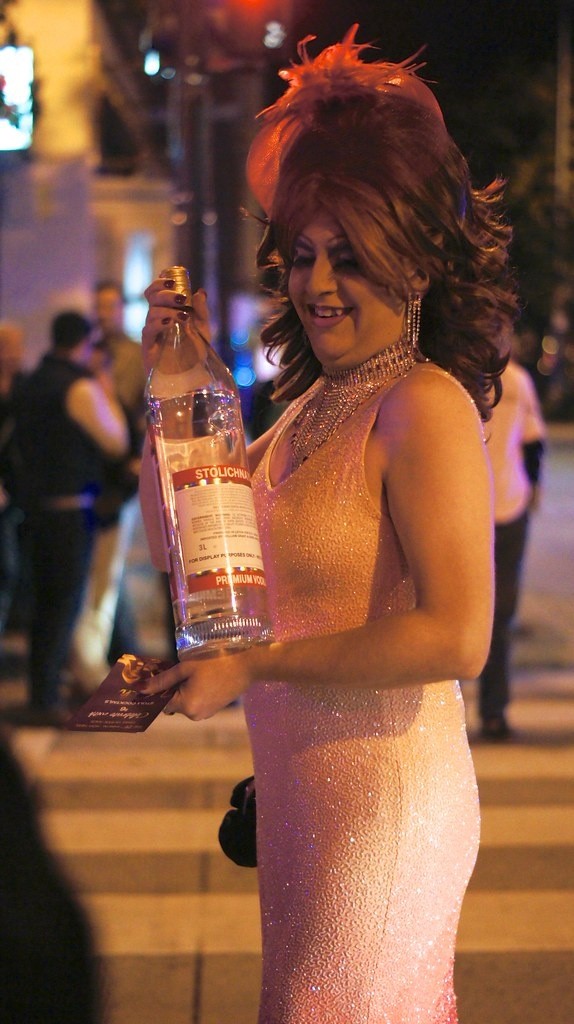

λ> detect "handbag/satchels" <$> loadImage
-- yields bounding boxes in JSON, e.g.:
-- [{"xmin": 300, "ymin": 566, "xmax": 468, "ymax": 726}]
[{"xmin": 218, "ymin": 775, "xmax": 258, "ymax": 868}]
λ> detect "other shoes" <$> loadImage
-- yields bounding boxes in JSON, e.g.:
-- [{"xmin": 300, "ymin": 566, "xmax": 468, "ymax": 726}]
[
  {"xmin": 22, "ymin": 705, "xmax": 73, "ymax": 725},
  {"xmin": 482, "ymin": 718, "xmax": 510, "ymax": 740}
]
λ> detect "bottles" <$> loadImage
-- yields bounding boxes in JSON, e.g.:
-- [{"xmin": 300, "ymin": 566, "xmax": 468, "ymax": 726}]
[{"xmin": 146, "ymin": 264, "xmax": 272, "ymax": 661}]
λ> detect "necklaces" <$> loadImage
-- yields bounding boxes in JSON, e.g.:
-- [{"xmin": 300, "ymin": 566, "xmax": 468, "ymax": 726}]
[{"xmin": 288, "ymin": 337, "xmax": 417, "ymax": 475}]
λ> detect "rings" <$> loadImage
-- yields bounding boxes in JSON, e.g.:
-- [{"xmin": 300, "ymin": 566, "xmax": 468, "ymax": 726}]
[{"xmin": 164, "ymin": 706, "xmax": 175, "ymax": 715}]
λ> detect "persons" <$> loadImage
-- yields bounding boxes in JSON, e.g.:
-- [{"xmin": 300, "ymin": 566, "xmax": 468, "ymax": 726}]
[{"xmin": 0, "ymin": 23, "xmax": 546, "ymax": 1024}]
